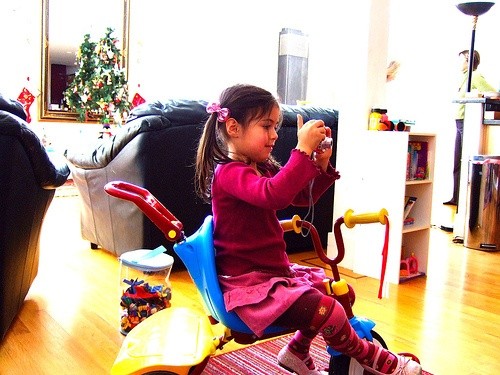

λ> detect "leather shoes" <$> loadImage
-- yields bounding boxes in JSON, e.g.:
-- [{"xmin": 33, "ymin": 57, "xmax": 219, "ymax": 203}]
[
  {"xmin": 361, "ymin": 345, "xmax": 422, "ymax": 375},
  {"xmin": 277, "ymin": 346, "xmax": 323, "ymax": 375}
]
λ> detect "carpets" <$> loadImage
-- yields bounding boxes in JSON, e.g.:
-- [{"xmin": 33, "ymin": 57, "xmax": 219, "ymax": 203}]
[{"xmin": 199, "ymin": 331, "xmax": 331, "ymax": 375}]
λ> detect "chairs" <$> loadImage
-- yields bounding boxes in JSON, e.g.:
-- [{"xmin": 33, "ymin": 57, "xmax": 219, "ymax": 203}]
[{"xmin": 173, "ymin": 215, "xmax": 296, "ymax": 345}]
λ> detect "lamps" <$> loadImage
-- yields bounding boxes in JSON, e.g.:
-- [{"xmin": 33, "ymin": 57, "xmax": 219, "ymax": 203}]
[{"xmin": 456, "ymin": 0, "xmax": 495, "ymax": 96}]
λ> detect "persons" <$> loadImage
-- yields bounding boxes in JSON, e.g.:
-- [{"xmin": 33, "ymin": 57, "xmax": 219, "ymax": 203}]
[
  {"xmin": 195, "ymin": 84, "xmax": 421, "ymax": 375},
  {"xmin": 443, "ymin": 50, "xmax": 497, "ymax": 205}
]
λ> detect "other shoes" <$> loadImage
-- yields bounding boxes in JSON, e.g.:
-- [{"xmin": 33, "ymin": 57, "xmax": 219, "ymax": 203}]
[{"xmin": 443, "ymin": 201, "xmax": 456, "ymax": 205}]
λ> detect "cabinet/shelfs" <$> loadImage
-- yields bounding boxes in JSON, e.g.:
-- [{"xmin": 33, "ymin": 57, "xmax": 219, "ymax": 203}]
[{"xmin": 326, "ymin": 130, "xmax": 437, "ymax": 299}]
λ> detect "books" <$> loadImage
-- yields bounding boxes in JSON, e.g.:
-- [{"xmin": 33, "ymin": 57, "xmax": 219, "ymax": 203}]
[
  {"xmin": 403, "ymin": 195, "xmax": 418, "ymax": 227},
  {"xmin": 406, "ymin": 140, "xmax": 431, "ymax": 181}
]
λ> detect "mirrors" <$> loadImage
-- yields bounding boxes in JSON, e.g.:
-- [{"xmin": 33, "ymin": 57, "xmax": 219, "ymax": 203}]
[{"xmin": 40, "ymin": 0, "xmax": 131, "ymax": 124}]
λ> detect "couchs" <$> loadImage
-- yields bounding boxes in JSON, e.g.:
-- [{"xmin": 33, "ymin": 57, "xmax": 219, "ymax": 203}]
[
  {"xmin": 65, "ymin": 100, "xmax": 340, "ymax": 276},
  {"xmin": 0, "ymin": 96, "xmax": 72, "ymax": 347}
]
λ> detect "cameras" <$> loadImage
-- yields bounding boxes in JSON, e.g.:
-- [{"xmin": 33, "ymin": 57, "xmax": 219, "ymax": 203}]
[{"xmin": 313, "ymin": 136, "xmax": 333, "ymax": 151}]
